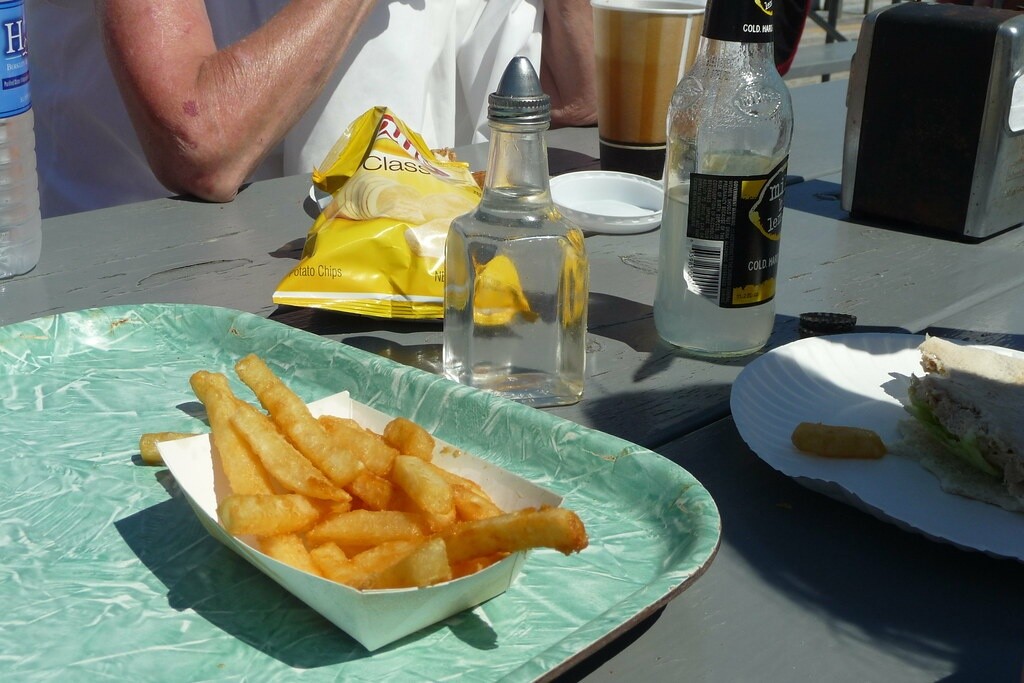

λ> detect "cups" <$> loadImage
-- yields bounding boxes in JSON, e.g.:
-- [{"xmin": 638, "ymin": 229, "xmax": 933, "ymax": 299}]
[{"xmin": 590, "ymin": 0, "xmax": 705, "ymax": 182}]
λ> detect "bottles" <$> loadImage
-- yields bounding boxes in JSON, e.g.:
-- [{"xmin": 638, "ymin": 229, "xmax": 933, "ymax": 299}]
[
  {"xmin": 654, "ymin": 0, "xmax": 792, "ymax": 357},
  {"xmin": 443, "ymin": 56, "xmax": 588, "ymax": 408},
  {"xmin": 0, "ymin": 0, "xmax": 42, "ymax": 278}
]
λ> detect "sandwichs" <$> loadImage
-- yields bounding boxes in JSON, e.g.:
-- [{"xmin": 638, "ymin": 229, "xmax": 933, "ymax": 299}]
[{"xmin": 886, "ymin": 335, "xmax": 1024, "ymax": 514}]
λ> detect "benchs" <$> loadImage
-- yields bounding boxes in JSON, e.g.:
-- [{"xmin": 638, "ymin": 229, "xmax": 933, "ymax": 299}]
[{"xmin": 781, "ymin": 41, "xmax": 858, "ymax": 79}]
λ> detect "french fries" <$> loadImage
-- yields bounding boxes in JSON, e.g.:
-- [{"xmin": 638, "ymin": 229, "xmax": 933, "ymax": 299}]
[
  {"xmin": 791, "ymin": 422, "xmax": 888, "ymax": 458},
  {"xmin": 138, "ymin": 353, "xmax": 589, "ymax": 593}
]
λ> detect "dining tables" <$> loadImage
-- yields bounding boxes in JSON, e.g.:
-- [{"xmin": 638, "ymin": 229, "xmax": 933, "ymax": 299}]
[{"xmin": 0, "ymin": 77, "xmax": 1024, "ymax": 683}]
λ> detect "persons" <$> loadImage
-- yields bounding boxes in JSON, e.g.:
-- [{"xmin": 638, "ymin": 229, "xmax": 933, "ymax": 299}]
[{"xmin": 24, "ymin": 0, "xmax": 597, "ymax": 219}]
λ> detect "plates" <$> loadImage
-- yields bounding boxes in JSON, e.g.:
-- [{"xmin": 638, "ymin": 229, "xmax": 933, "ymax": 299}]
[{"xmin": 729, "ymin": 333, "xmax": 1024, "ymax": 560}]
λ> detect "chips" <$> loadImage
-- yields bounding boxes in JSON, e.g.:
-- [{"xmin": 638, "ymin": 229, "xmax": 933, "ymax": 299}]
[{"xmin": 337, "ymin": 174, "xmax": 477, "ymax": 262}]
